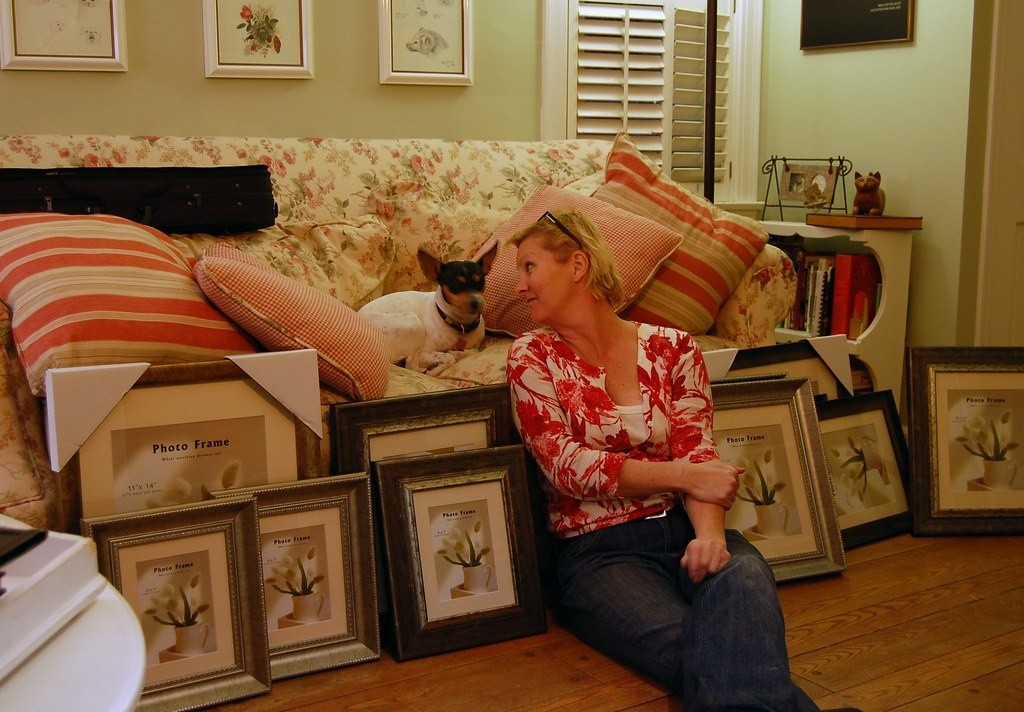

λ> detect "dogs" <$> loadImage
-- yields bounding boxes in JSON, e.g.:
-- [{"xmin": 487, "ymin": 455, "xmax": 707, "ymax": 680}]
[{"xmin": 357, "ymin": 239, "xmax": 499, "ymax": 380}]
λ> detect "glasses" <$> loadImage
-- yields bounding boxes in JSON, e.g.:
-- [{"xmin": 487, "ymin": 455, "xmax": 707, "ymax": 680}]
[{"xmin": 537, "ymin": 210, "xmax": 584, "ymax": 251}]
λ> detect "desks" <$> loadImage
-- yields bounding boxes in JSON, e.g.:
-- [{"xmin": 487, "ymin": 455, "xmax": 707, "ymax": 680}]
[{"xmin": 0, "ymin": 511, "xmax": 148, "ymax": 712}]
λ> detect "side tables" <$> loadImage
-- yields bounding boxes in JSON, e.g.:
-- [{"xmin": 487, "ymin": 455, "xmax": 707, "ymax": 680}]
[{"xmin": 753, "ymin": 214, "xmax": 923, "ymax": 414}]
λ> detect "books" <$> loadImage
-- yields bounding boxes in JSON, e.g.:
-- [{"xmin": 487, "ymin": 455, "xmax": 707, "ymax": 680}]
[{"xmin": 775, "ymin": 250, "xmax": 880, "ymax": 340}]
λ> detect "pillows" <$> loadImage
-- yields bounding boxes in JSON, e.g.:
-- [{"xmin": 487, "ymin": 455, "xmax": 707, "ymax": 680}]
[
  {"xmin": 195, "ymin": 243, "xmax": 387, "ymax": 402},
  {"xmin": 462, "ymin": 186, "xmax": 683, "ymax": 349},
  {"xmin": 0, "ymin": 210, "xmax": 269, "ymax": 419},
  {"xmin": 591, "ymin": 132, "xmax": 769, "ymax": 335}
]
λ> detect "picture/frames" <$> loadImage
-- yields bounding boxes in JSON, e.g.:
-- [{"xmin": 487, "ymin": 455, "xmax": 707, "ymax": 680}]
[
  {"xmin": 800, "ymin": 0, "xmax": 914, "ymax": 50},
  {"xmin": 902, "ymin": 347, "xmax": 1024, "ymax": 538},
  {"xmin": 379, "ymin": 0, "xmax": 474, "ymax": 91},
  {"xmin": 710, "ymin": 378, "xmax": 846, "ymax": 586},
  {"xmin": 0, "ymin": 0, "xmax": 128, "ymax": 73},
  {"xmin": 327, "ymin": 381, "xmax": 543, "ymax": 651},
  {"xmin": 691, "ymin": 334, "xmax": 861, "ymax": 457},
  {"xmin": 203, "ymin": 0, "xmax": 315, "ymax": 81},
  {"xmin": 210, "ymin": 474, "xmax": 380, "ymax": 678},
  {"xmin": 375, "ymin": 444, "xmax": 548, "ymax": 664},
  {"xmin": 816, "ymin": 390, "xmax": 912, "ymax": 544},
  {"xmin": 41, "ymin": 349, "xmax": 318, "ymax": 560},
  {"xmin": 81, "ymin": 492, "xmax": 271, "ymax": 712}
]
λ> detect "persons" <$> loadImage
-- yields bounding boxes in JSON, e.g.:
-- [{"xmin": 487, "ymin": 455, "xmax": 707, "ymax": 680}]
[{"xmin": 502, "ymin": 210, "xmax": 864, "ymax": 712}]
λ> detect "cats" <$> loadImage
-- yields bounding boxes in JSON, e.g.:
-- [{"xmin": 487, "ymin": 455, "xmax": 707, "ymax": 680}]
[{"xmin": 853, "ymin": 170, "xmax": 885, "ymax": 216}]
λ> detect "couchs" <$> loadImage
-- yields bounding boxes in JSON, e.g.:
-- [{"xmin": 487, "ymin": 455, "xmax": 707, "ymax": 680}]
[{"xmin": 0, "ymin": 134, "xmax": 794, "ymax": 534}]
